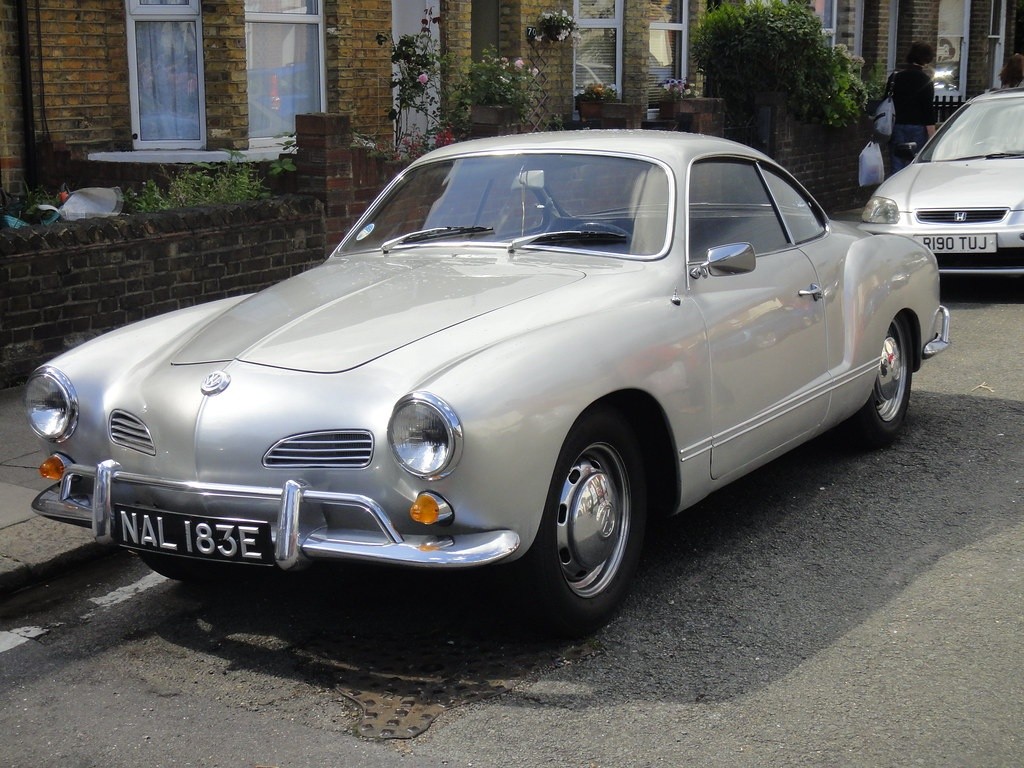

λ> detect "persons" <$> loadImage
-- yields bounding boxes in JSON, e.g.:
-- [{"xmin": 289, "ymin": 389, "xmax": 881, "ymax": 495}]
[
  {"xmin": 869, "ymin": 40, "xmax": 939, "ymax": 177},
  {"xmin": 998, "ymin": 52, "xmax": 1024, "ymax": 88}
]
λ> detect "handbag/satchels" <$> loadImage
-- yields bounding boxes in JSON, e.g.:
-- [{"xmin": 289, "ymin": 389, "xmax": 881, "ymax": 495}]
[
  {"xmin": 872, "ymin": 96, "xmax": 896, "ymax": 144},
  {"xmin": 859, "ymin": 139, "xmax": 884, "ymax": 188}
]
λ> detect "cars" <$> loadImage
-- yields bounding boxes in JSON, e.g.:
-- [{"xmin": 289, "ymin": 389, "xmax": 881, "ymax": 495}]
[
  {"xmin": 859, "ymin": 88, "xmax": 1024, "ymax": 305},
  {"xmin": 27, "ymin": 125, "xmax": 949, "ymax": 637}
]
